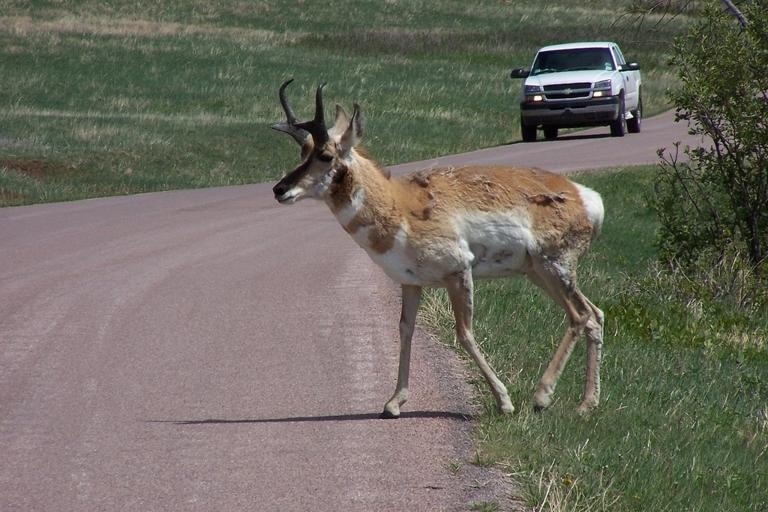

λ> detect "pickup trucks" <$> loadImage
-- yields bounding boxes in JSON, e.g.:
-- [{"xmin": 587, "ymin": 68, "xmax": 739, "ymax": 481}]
[{"xmin": 510, "ymin": 40, "xmax": 645, "ymax": 141}]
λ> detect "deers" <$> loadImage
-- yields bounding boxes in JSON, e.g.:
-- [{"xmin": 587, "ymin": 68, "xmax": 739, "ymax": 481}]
[{"xmin": 270, "ymin": 77, "xmax": 605, "ymax": 419}]
